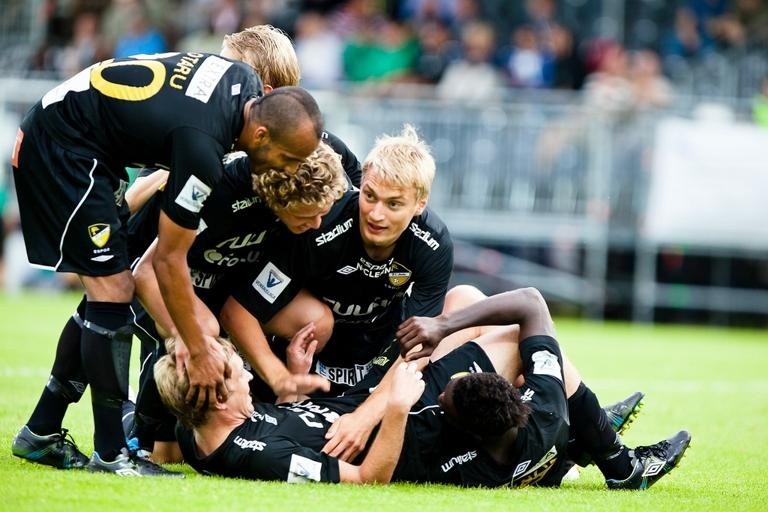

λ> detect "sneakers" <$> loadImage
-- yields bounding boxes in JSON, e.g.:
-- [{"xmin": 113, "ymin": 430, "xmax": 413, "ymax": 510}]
[
  {"xmin": 12, "ymin": 426, "xmax": 184, "ymax": 478},
  {"xmin": 571, "ymin": 392, "xmax": 644, "ymax": 468},
  {"xmin": 606, "ymin": 430, "xmax": 691, "ymax": 490}
]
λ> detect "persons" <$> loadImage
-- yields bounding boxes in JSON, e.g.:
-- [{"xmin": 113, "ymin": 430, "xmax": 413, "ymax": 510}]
[
  {"xmin": 11, "ymin": 25, "xmax": 691, "ymax": 489},
  {"xmin": 1, "ymin": 2, "xmax": 768, "ymax": 120}
]
